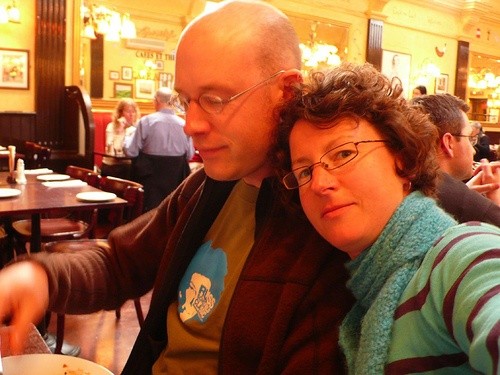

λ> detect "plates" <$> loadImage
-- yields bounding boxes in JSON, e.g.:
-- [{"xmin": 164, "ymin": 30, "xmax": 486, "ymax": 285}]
[
  {"xmin": 0, "ymin": 188, "xmax": 20, "ymax": 197},
  {"xmin": 76, "ymin": 191, "xmax": 117, "ymax": 202},
  {"xmin": 0, "ymin": 355, "xmax": 117, "ymax": 375},
  {"xmin": 36, "ymin": 174, "xmax": 70, "ymax": 181}
]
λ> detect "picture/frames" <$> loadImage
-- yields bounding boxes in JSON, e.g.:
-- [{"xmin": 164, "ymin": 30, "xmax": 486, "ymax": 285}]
[
  {"xmin": 113, "ymin": 82, "xmax": 133, "ymax": 99},
  {"xmin": 154, "ymin": 60, "xmax": 165, "ymax": 70},
  {"xmin": 382, "ymin": 50, "xmax": 414, "ymax": 98},
  {"xmin": 134, "ymin": 79, "xmax": 156, "ymax": 100},
  {"xmin": 158, "ymin": 72, "xmax": 174, "ymax": 91},
  {"xmin": 433, "ymin": 71, "xmax": 449, "ymax": 95},
  {"xmin": 487, "ymin": 105, "xmax": 499, "ymax": 123},
  {"xmin": 120, "ymin": 66, "xmax": 133, "ymax": 80},
  {"xmin": 110, "ymin": 69, "xmax": 119, "ymax": 80},
  {"xmin": 0, "ymin": 47, "xmax": 32, "ymax": 90}
]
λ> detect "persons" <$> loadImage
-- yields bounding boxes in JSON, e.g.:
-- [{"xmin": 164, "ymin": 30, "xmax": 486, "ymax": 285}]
[
  {"xmin": 97, "ymin": 99, "xmax": 141, "ymax": 226},
  {"xmin": 123, "ymin": 87, "xmax": 195, "ymax": 214},
  {"xmin": 469, "ymin": 122, "xmax": 489, "ymax": 163},
  {"xmin": 1, "ymin": 0, "xmax": 351, "ymax": 375},
  {"xmin": 407, "ymin": 93, "xmax": 500, "ymax": 228},
  {"xmin": 263, "ymin": 60, "xmax": 500, "ymax": 375},
  {"xmin": 411, "ymin": 85, "xmax": 427, "ymax": 100}
]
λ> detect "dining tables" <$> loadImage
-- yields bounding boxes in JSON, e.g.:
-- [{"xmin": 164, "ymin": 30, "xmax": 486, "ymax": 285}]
[{"xmin": 1, "ymin": 168, "xmax": 128, "ymax": 265}]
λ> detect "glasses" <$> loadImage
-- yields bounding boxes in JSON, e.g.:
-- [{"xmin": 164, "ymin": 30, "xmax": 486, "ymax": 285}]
[
  {"xmin": 451, "ymin": 133, "xmax": 478, "ymax": 147},
  {"xmin": 168, "ymin": 70, "xmax": 285, "ymax": 114},
  {"xmin": 281, "ymin": 139, "xmax": 393, "ymax": 190}
]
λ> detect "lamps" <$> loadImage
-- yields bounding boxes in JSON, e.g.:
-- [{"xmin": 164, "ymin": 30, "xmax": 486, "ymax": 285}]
[
  {"xmin": 297, "ymin": 22, "xmax": 342, "ymax": 68},
  {"xmin": 468, "ymin": 65, "xmax": 500, "ymax": 91},
  {"xmin": 80, "ymin": 2, "xmax": 138, "ymax": 44},
  {"xmin": 1, "ymin": 1, "xmax": 20, "ymax": 26}
]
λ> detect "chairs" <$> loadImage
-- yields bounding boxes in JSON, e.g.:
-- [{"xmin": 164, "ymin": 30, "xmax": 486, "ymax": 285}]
[
  {"xmin": 37, "ymin": 177, "xmax": 148, "ymax": 353},
  {"xmin": 20, "ymin": 140, "xmax": 48, "ymax": 168},
  {"xmin": 11, "ymin": 165, "xmax": 95, "ymax": 255}
]
